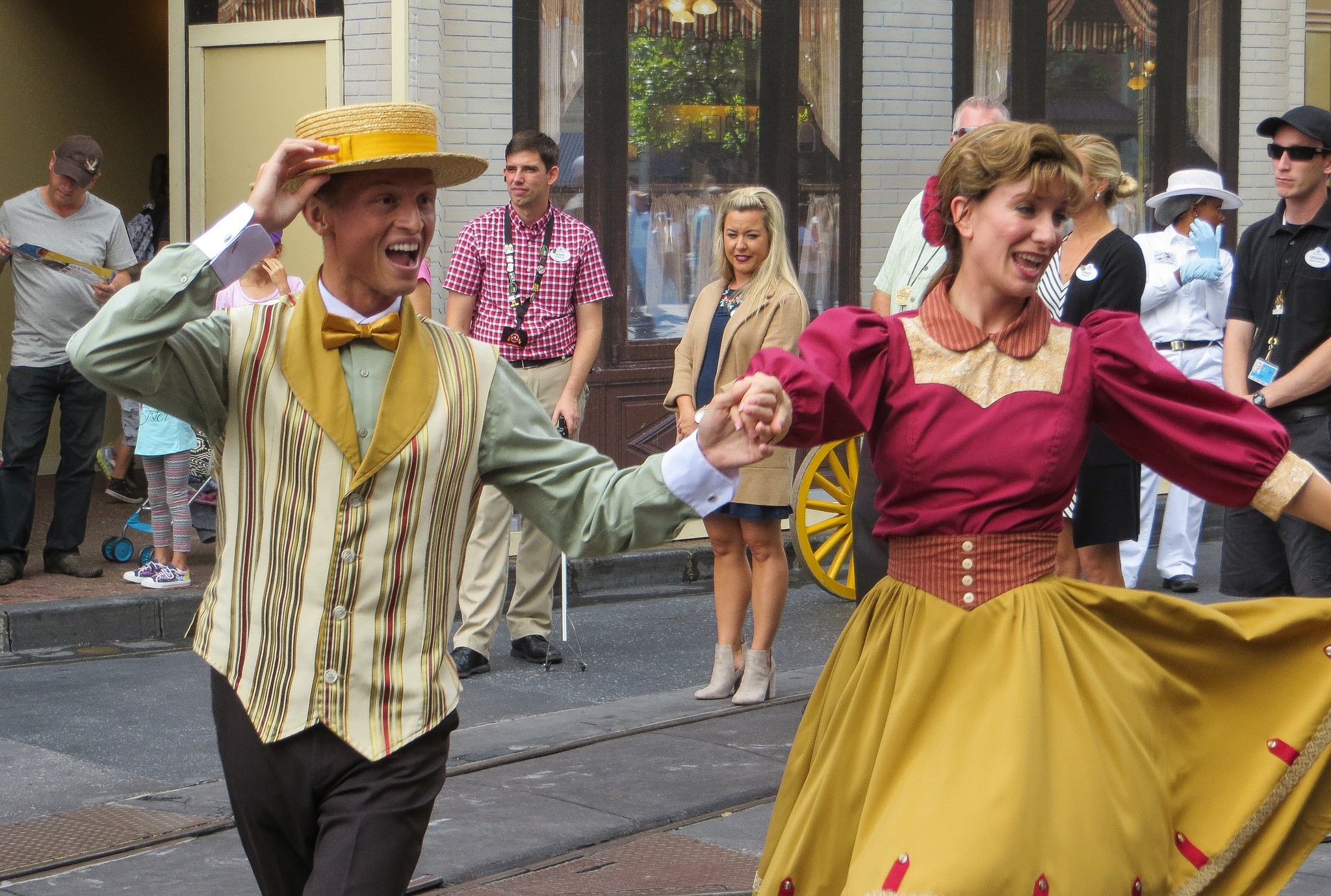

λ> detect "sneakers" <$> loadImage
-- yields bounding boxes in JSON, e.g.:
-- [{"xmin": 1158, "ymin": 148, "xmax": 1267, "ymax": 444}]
[
  {"xmin": 140, "ymin": 564, "xmax": 191, "ymax": 589},
  {"xmin": 42, "ymin": 551, "xmax": 104, "ymax": 579},
  {"xmin": 1162, "ymin": 573, "xmax": 1200, "ymax": 593},
  {"xmin": 95, "ymin": 443, "xmax": 117, "ymax": 481},
  {"xmin": 123, "ymin": 560, "xmax": 171, "ymax": 584},
  {"xmin": 0, "ymin": 558, "xmax": 23, "ymax": 587},
  {"xmin": 105, "ymin": 477, "xmax": 145, "ymax": 504}
]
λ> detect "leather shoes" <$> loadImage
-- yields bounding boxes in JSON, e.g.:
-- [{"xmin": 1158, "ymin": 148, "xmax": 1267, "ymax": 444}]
[
  {"xmin": 448, "ymin": 646, "xmax": 491, "ymax": 680},
  {"xmin": 509, "ymin": 633, "xmax": 562, "ymax": 664}
]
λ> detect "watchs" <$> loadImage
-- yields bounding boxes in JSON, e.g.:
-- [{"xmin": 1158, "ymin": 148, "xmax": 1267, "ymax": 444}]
[{"xmin": 1252, "ymin": 391, "xmax": 1270, "ymax": 412}]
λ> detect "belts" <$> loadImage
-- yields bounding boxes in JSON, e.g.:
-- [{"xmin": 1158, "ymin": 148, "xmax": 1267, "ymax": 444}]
[
  {"xmin": 508, "ymin": 353, "xmax": 576, "ymax": 371},
  {"xmin": 1261, "ymin": 403, "xmax": 1331, "ymax": 422},
  {"xmin": 1147, "ymin": 336, "xmax": 1221, "ymax": 355}
]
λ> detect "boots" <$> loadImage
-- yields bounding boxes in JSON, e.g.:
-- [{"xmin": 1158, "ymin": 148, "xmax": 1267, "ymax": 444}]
[
  {"xmin": 731, "ymin": 648, "xmax": 776, "ymax": 705},
  {"xmin": 695, "ymin": 641, "xmax": 750, "ymax": 699}
]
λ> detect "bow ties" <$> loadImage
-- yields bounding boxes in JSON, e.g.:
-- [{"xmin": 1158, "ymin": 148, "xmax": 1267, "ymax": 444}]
[{"xmin": 319, "ymin": 311, "xmax": 400, "ymax": 353}]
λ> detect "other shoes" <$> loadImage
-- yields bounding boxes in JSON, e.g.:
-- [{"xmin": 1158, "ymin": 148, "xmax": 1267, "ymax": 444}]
[{"xmin": 627, "ymin": 312, "xmax": 654, "ymax": 327}]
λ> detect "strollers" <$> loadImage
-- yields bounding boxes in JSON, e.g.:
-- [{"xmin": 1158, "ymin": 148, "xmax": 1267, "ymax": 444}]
[{"xmin": 103, "ymin": 473, "xmax": 217, "ymax": 565}]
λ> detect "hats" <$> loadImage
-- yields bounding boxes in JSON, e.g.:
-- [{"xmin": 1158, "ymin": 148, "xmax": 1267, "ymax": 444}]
[
  {"xmin": 706, "ymin": 186, "xmax": 724, "ymax": 196},
  {"xmin": 249, "ymin": 103, "xmax": 487, "ymax": 194},
  {"xmin": 1145, "ymin": 168, "xmax": 1244, "ymax": 211},
  {"xmin": 627, "ymin": 189, "xmax": 649, "ymax": 200},
  {"xmin": 1255, "ymin": 106, "xmax": 1331, "ymax": 145}
]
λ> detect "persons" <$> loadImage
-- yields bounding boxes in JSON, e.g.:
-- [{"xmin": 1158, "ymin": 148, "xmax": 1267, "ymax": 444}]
[
  {"xmin": 97, "ymin": 431, "xmax": 125, "ymax": 480},
  {"xmin": 105, "ymin": 150, "xmax": 171, "ymax": 503},
  {"xmin": 628, "ymin": 174, "xmax": 840, "ymax": 328},
  {"xmin": 444, "ymin": 128, "xmax": 611, "ymax": 680},
  {"xmin": 664, "ymin": 188, "xmax": 810, "ymax": 708},
  {"xmin": 0, "ymin": 135, "xmax": 139, "ymax": 585},
  {"xmin": 214, "ymin": 227, "xmax": 305, "ymax": 312},
  {"xmin": 1033, "ymin": 133, "xmax": 1148, "ymax": 594},
  {"xmin": 1120, "ymin": 168, "xmax": 1233, "ymax": 595},
  {"xmin": 121, "ymin": 398, "xmax": 195, "ymax": 590},
  {"xmin": 1220, "ymin": 106, "xmax": 1331, "ymax": 602},
  {"xmin": 408, "ymin": 257, "xmax": 435, "ymax": 321},
  {"xmin": 68, "ymin": 102, "xmax": 780, "ymax": 895},
  {"xmin": 853, "ymin": 97, "xmax": 1012, "ymax": 605},
  {"xmin": 153, "ymin": 158, "xmax": 171, "ymax": 256},
  {"xmin": 725, "ymin": 121, "xmax": 1331, "ymax": 895}
]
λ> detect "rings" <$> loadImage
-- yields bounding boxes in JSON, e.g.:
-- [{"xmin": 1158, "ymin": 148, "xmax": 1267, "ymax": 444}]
[{"xmin": 575, "ymin": 426, "xmax": 578, "ymax": 428}]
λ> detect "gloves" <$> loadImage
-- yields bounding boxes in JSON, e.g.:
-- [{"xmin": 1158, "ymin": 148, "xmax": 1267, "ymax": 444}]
[
  {"xmin": 1188, "ymin": 217, "xmax": 1223, "ymax": 262},
  {"xmin": 1176, "ymin": 255, "xmax": 1222, "ymax": 288}
]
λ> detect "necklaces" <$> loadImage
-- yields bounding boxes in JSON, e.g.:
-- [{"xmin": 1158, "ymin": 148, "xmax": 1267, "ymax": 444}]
[
  {"xmin": 720, "ymin": 277, "xmax": 744, "ymax": 309},
  {"xmin": 1272, "ymin": 232, "xmax": 1316, "ymax": 318},
  {"xmin": 1064, "ymin": 221, "xmax": 1113, "ymax": 275},
  {"xmin": 899, "ymin": 238, "xmax": 943, "ymax": 312}
]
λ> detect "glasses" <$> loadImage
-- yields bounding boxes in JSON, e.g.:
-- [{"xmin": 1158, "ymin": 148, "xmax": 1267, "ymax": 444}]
[
  {"xmin": 1265, "ymin": 142, "xmax": 1330, "ymax": 162},
  {"xmin": 951, "ymin": 126, "xmax": 978, "ymax": 139}
]
